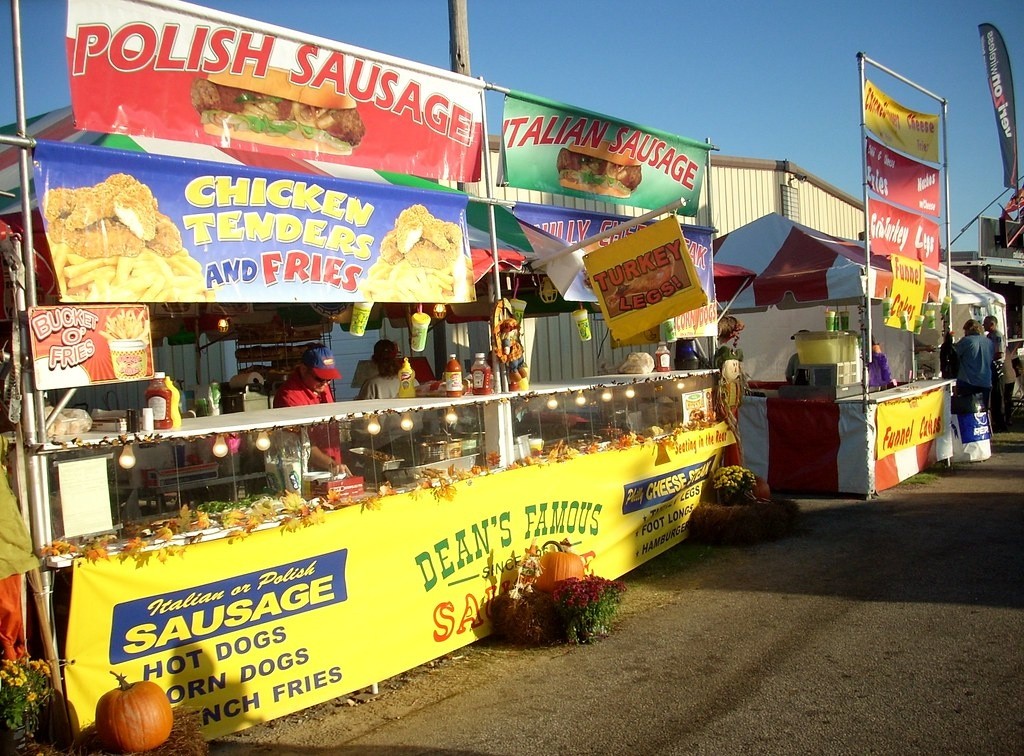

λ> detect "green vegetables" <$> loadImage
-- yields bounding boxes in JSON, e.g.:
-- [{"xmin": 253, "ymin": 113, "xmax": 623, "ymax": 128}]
[{"xmin": 194, "ymin": 492, "xmax": 254, "ymax": 514}]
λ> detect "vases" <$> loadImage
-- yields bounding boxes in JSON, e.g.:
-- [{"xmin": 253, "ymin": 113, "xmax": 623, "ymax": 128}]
[{"xmin": 716, "ymin": 487, "xmax": 738, "ymax": 506}]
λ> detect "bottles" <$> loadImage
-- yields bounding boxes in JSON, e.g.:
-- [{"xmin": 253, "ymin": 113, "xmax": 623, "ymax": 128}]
[
  {"xmin": 210, "ymin": 383, "xmax": 221, "ymax": 416},
  {"xmin": 444, "ymin": 353, "xmax": 463, "ymax": 398},
  {"xmin": 144, "ymin": 372, "xmax": 174, "ymax": 430},
  {"xmin": 470, "ymin": 353, "xmax": 492, "ymax": 395},
  {"xmin": 681, "ymin": 348, "xmax": 699, "ymax": 370},
  {"xmin": 163, "ymin": 376, "xmax": 181, "ymax": 428},
  {"xmin": 655, "ymin": 342, "xmax": 672, "ymax": 372},
  {"xmin": 397, "ymin": 357, "xmax": 416, "ymax": 399}
]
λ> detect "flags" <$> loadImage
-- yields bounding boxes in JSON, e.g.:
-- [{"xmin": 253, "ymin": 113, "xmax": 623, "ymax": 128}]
[{"xmin": 978, "ymin": 23, "xmax": 1020, "ymax": 189}]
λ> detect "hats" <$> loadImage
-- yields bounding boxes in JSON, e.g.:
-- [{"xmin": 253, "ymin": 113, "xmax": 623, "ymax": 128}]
[
  {"xmin": 301, "ymin": 345, "xmax": 343, "ymax": 380},
  {"xmin": 373, "ymin": 339, "xmax": 397, "ymax": 360}
]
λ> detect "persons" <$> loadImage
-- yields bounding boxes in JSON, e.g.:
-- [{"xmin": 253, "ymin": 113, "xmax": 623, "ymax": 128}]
[
  {"xmin": 272, "ymin": 345, "xmax": 354, "ymax": 479},
  {"xmin": 914, "ymin": 339, "xmax": 935, "ymax": 355},
  {"xmin": 357, "ymin": 336, "xmax": 424, "ymax": 467},
  {"xmin": 982, "ymin": 315, "xmax": 1007, "ymax": 424},
  {"xmin": 951, "ymin": 318, "xmax": 994, "ymax": 418},
  {"xmin": 785, "ymin": 330, "xmax": 811, "ymax": 385}
]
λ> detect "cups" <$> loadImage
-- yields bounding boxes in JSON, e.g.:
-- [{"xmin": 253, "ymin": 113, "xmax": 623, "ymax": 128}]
[
  {"xmin": 899, "ymin": 311, "xmax": 907, "ymax": 331},
  {"xmin": 570, "ymin": 309, "xmax": 591, "ymax": 341},
  {"xmin": 510, "ymin": 298, "xmax": 527, "ymax": 324},
  {"xmin": 824, "ymin": 310, "xmax": 835, "ymax": 332},
  {"xmin": 410, "ymin": 312, "xmax": 431, "ymax": 352},
  {"xmin": 660, "ymin": 318, "xmax": 677, "ymax": 343},
  {"xmin": 834, "ymin": 310, "xmax": 839, "ymax": 332},
  {"xmin": 349, "ymin": 302, "xmax": 374, "ymax": 337},
  {"xmin": 528, "ymin": 438, "xmax": 544, "ymax": 457},
  {"xmin": 940, "ymin": 296, "xmax": 952, "ymax": 315},
  {"xmin": 840, "ymin": 310, "xmax": 849, "ymax": 332},
  {"xmin": 925, "ymin": 310, "xmax": 936, "ymax": 330},
  {"xmin": 912, "ymin": 315, "xmax": 925, "ymax": 335},
  {"xmin": 882, "ymin": 296, "xmax": 892, "ymax": 318}
]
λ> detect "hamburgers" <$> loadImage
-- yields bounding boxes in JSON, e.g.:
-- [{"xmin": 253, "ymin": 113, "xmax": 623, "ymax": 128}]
[
  {"xmin": 190, "ymin": 61, "xmax": 365, "ymax": 155},
  {"xmin": 556, "ymin": 137, "xmax": 643, "ymax": 198}
]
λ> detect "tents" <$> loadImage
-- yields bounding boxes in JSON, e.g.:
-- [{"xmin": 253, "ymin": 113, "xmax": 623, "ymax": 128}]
[
  {"xmin": 937, "ymin": 262, "xmax": 1007, "ymax": 350},
  {"xmin": 712, "ymin": 212, "xmax": 953, "ymax": 502}
]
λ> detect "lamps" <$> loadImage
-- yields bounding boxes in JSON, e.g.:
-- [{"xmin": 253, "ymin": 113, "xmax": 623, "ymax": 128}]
[{"xmin": 790, "ymin": 173, "xmax": 807, "ymax": 183}]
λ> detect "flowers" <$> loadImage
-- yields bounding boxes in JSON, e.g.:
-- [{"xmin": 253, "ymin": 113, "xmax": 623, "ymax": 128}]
[{"xmin": 713, "ymin": 465, "xmax": 757, "ymax": 493}]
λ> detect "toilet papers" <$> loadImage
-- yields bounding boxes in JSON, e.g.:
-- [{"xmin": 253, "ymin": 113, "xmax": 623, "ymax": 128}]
[{"xmin": 228, "ymin": 372, "xmax": 265, "ymax": 388}]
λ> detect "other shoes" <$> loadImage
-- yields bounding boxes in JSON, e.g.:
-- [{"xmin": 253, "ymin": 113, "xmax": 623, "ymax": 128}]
[{"xmin": 991, "ymin": 418, "xmax": 1012, "ymax": 433}]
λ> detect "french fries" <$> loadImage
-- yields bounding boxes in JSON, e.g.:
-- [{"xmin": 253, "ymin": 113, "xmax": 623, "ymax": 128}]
[
  {"xmin": 98, "ymin": 309, "xmax": 149, "ymax": 341},
  {"xmin": 359, "ymin": 257, "xmax": 476, "ymax": 303},
  {"xmin": 49, "ymin": 243, "xmax": 207, "ymax": 303}
]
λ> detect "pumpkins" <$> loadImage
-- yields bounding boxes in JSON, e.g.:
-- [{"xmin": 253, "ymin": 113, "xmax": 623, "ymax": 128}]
[
  {"xmin": 750, "ymin": 476, "xmax": 770, "ymax": 500},
  {"xmin": 95, "ymin": 671, "xmax": 173, "ymax": 754},
  {"xmin": 534, "ymin": 541, "xmax": 584, "ymax": 592}
]
